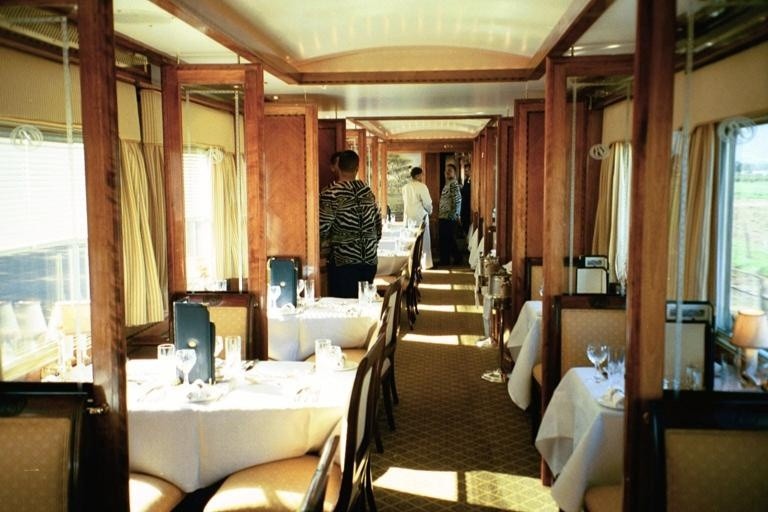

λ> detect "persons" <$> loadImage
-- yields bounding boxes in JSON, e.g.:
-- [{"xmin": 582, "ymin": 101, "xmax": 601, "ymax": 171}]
[
  {"xmin": 319, "ymin": 150, "xmax": 382, "ymax": 298},
  {"xmin": 320, "ymin": 153, "xmax": 341, "ymax": 193},
  {"xmin": 437, "ymin": 164, "xmax": 466, "ymax": 266},
  {"xmin": 401, "ymin": 167, "xmax": 434, "ymax": 269},
  {"xmin": 461, "ymin": 164, "xmax": 471, "ymax": 254}
]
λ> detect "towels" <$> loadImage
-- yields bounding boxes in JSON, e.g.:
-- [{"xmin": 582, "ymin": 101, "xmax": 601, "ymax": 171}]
[{"xmin": 605, "ymin": 384, "xmax": 625, "ymax": 408}]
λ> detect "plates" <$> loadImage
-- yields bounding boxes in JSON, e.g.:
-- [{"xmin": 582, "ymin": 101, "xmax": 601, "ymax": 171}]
[{"xmin": 594, "ymin": 390, "xmax": 624, "ymax": 410}]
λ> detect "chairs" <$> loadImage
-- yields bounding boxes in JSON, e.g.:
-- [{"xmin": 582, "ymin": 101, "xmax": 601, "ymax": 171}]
[
  {"xmin": 583, "ymin": 368, "xmax": 762, "ymax": 512},
  {"xmin": 584, "ymin": 390, "xmax": 768, "ymax": 506},
  {"xmin": 374, "ymin": 235, "xmax": 422, "ymax": 331},
  {"xmin": 129, "ymin": 471, "xmax": 184, "ymax": 512},
  {"xmin": 368, "ymin": 307, "xmax": 390, "ymax": 453},
  {"xmin": 416, "ymin": 221, "xmax": 426, "ymax": 284},
  {"xmin": 305, "ymin": 279, "xmax": 401, "ymax": 431},
  {"xmin": 577, "ymin": 267, "xmax": 606, "ymax": 294},
  {"xmin": 422, "ymin": 214, "xmax": 427, "ymax": 221},
  {"xmin": 300, "ymin": 435, "xmax": 339, "ymax": 512},
  {"xmin": 630, "ymin": 401, "xmax": 768, "ymax": 512},
  {"xmin": 0, "ymin": 382, "xmax": 122, "ymax": 512},
  {"xmin": 526, "ymin": 257, "xmax": 578, "ymax": 301},
  {"xmin": 665, "ymin": 304, "xmax": 712, "ymax": 390},
  {"xmin": 479, "ymin": 217, "xmax": 482, "ymax": 243},
  {"xmin": 532, "ymin": 363, "xmax": 542, "ymax": 384},
  {"xmin": 549, "ymin": 294, "xmax": 626, "ymax": 403},
  {"xmin": 486, "ymin": 227, "xmax": 496, "ymax": 256},
  {"xmin": 413, "ymin": 228, "xmax": 425, "ymax": 303},
  {"xmin": 205, "ymin": 332, "xmax": 385, "ymax": 512},
  {"xmin": 188, "ymin": 292, "xmax": 254, "ymax": 360}
]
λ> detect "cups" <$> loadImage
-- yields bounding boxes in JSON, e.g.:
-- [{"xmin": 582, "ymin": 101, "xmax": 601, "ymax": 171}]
[
  {"xmin": 268, "ymin": 285, "xmax": 281, "ymax": 314},
  {"xmin": 303, "ymin": 278, "xmax": 316, "ymax": 307},
  {"xmin": 224, "ymin": 335, "xmax": 242, "ymax": 374},
  {"xmin": 369, "ymin": 284, "xmax": 378, "ymax": 305},
  {"xmin": 412, "ymin": 221, "xmax": 416, "ymax": 230},
  {"xmin": 314, "ymin": 338, "xmax": 332, "ymax": 376},
  {"xmin": 176, "ymin": 348, "xmax": 198, "ymax": 395},
  {"xmin": 408, "ymin": 220, "xmax": 412, "ymax": 229},
  {"xmin": 296, "ymin": 278, "xmax": 307, "ymax": 306},
  {"xmin": 358, "ymin": 281, "xmax": 371, "ymax": 309},
  {"xmin": 720, "ymin": 352, "xmax": 742, "ymax": 391},
  {"xmin": 605, "ymin": 345, "xmax": 626, "ymax": 390},
  {"xmin": 332, "ymin": 344, "xmax": 343, "ymax": 375},
  {"xmin": 586, "ymin": 342, "xmax": 609, "ymax": 386},
  {"xmin": 215, "ymin": 335, "xmax": 224, "ymax": 377},
  {"xmin": 399, "ymin": 227, "xmax": 406, "ymax": 239},
  {"xmin": 390, "ymin": 214, "xmax": 395, "ymax": 223},
  {"xmin": 394, "ymin": 238, "xmax": 400, "ymax": 251},
  {"xmin": 156, "ymin": 343, "xmax": 176, "ymax": 389}
]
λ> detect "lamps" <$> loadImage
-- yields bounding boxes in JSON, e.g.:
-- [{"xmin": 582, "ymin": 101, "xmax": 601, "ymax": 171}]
[
  {"xmin": 729, "ymin": 311, "xmax": 768, "ymax": 386},
  {"xmin": 49, "ymin": 301, "xmax": 91, "ymax": 367},
  {"xmin": 15, "ymin": 300, "xmax": 47, "ymax": 340},
  {"xmin": 0, "ymin": 303, "xmax": 20, "ymax": 381}
]
